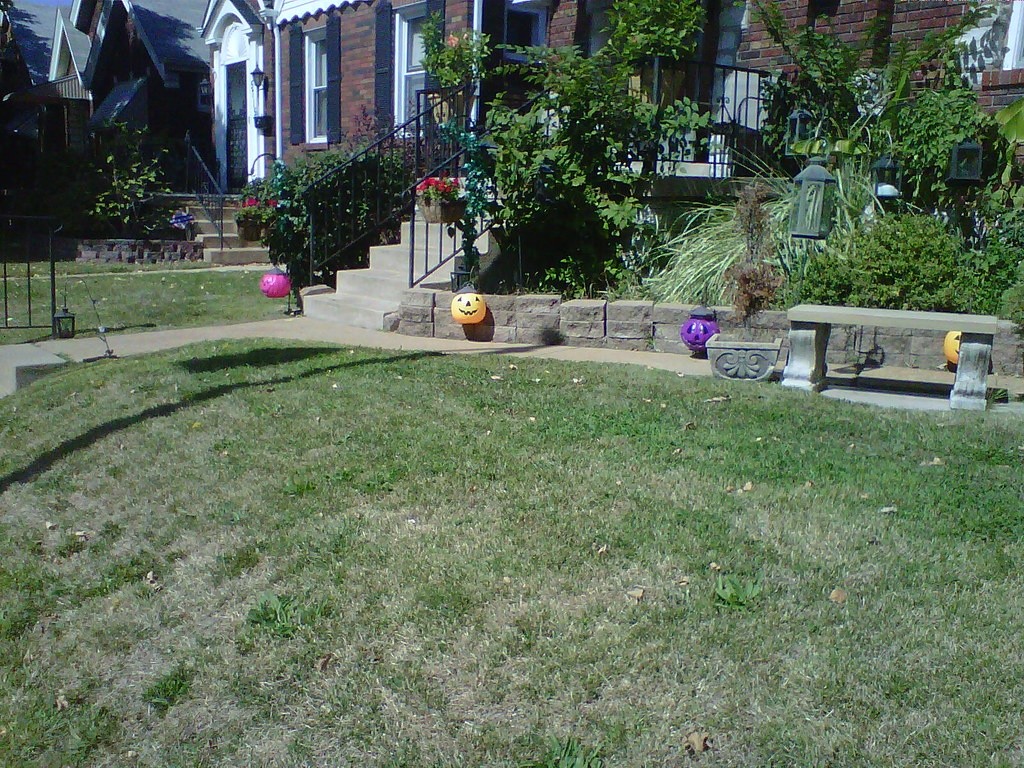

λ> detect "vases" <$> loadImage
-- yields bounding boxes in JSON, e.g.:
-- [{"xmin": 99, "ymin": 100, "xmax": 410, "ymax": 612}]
[
  {"xmin": 234, "ymin": 218, "xmax": 269, "ymax": 240},
  {"xmin": 417, "ymin": 204, "xmax": 456, "ymax": 224}
]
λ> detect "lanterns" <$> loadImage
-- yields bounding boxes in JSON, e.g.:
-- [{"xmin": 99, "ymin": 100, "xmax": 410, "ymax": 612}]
[
  {"xmin": 452, "ymin": 294, "xmax": 488, "ymax": 325},
  {"xmin": 259, "ymin": 274, "xmax": 292, "ymax": 297},
  {"xmin": 944, "ymin": 331, "xmax": 963, "ymax": 367},
  {"xmin": 680, "ymin": 315, "xmax": 719, "ymax": 354}
]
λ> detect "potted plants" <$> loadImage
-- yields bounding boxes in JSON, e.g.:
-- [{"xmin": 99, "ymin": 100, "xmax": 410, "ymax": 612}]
[{"xmin": 702, "ymin": 166, "xmax": 784, "ymax": 384}]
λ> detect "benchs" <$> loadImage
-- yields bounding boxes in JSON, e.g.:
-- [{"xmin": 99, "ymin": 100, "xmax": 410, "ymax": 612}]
[{"xmin": 780, "ymin": 300, "xmax": 1007, "ymax": 414}]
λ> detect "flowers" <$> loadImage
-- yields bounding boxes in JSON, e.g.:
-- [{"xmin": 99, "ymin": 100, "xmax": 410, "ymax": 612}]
[
  {"xmin": 413, "ymin": 176, "xmax": 461, "ymax": 206},
  {"xmin": 231, "ymin": 199, "xmax": 275, "ymax": 224}
]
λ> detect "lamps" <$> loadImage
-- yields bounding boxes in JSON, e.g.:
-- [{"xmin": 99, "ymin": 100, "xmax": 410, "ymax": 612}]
[
  {"xmin": 54, "ymin": 275, "xmax": 119, "ymax": 361},
  {"xmin": 246, "ymin": 61, "xmax": 272, "ymax": 133}
]
